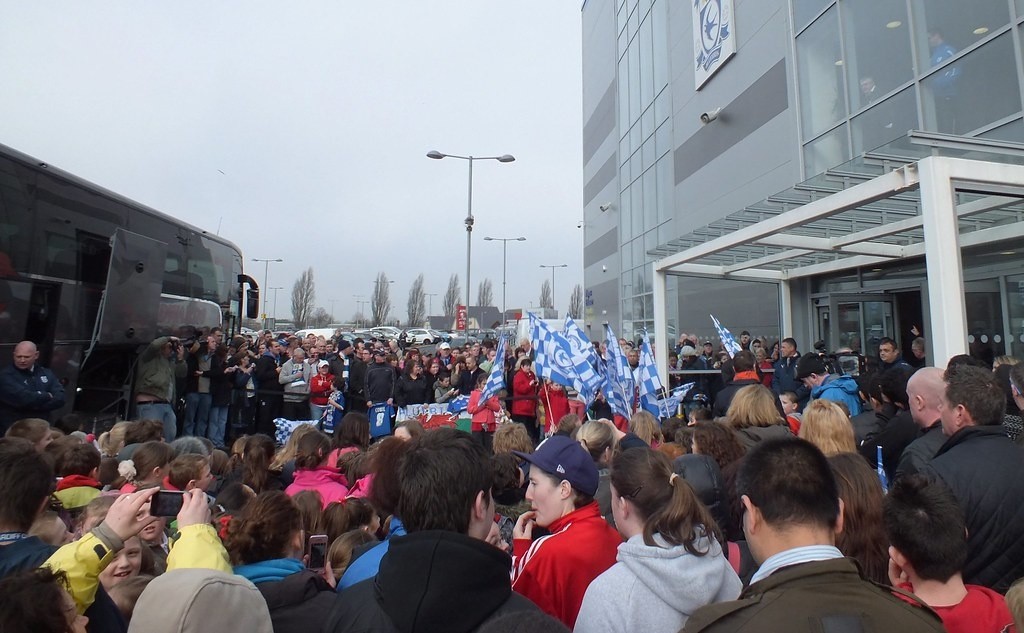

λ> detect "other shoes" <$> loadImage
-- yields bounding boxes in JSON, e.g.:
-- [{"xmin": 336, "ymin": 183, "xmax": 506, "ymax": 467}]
[{"xmin": 218, "ymin": 446, "xmax": 230, "ymax": 450}]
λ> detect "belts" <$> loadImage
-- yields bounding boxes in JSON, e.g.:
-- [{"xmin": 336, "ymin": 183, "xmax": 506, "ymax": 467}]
[{"xmin": 137, "ymin": 400, "xmax": 169, "ymax": 405}]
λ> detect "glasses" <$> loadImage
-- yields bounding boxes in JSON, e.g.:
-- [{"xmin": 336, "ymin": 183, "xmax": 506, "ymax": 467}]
[{"xmin": 493, "ymin": 536, "xmax": 506, "ymax": 548}]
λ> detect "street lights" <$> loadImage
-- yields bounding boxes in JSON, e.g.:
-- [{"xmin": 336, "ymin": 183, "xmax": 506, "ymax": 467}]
[
  {"xmin": 539, "ymin": 265, "xmax": 567, "ymax": 325},
  {"xmin": 251, "ymin": 258, "xmax": 284, "ymax": 336},
  {"xmin": 422, "ymin": 292, "xmax": 437, "ymax": 330},
  {"xmin": 426, "ymin": 149, "xmax": 515, "ymax": 347},
  {"xmin": 269, "ymin": 287, "xmax": 283, "ymax": 332},
  {"xmin": 327, "ymin": 294, "xmax": 370, "ymax": 328},
  {"xmin": 483, "ymin": 236, "xmax": 527, "ymax": 334},
  {"xmin": 373, "ymin": 280, "xmax": 393, "ymax": 327}
]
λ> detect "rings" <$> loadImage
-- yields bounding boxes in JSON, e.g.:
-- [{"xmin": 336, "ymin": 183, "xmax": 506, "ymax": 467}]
[{"xmin": 125, "ymin": 495, "xmax": 131, "ymax": 500}]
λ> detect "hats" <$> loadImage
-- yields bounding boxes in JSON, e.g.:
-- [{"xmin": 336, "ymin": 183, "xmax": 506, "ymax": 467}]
[
  {"xmin": 703, "ymin": 341, "xmax": 712, "ymax": 347},
  {"xmin": 681, "ymin": 346, "xmax": 695, "ymax": 356},
  {"xmin": 293, "ymin": 348, "xmax": 305, "ymax": 356},
  {"xmin": 229, "ymin": 337, "xmax": 246, "ymax": 353},
  {"xmin": 422, "ymin": 352, "xmax": 432, "ymax": 357},
  {"xmin": 440, "ymin": 343, "xmax": 450, "ymax": 349},
  {"xmin": 318, "ymin": 361, "xmax": 329, "ymax": 368},
  {"xmin": 511, "ymin": 436, "xmax": 599, "ymax": 496},
  {"xmin": 339, "ymin": 341, "xmax": 350, "ymax": 350},
  {"xmin": 798, "ymin": 352, "xmax": 826, "ymax": 378}
]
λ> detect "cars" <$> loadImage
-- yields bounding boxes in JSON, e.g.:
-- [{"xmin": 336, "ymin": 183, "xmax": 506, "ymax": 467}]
[
  {"xmin": 293, "ymin": 327, "xmax": 403, "ymax": 348},
  {"xmin": 401, "ymin": 329, "xmax": 455, "ymax": 345}
]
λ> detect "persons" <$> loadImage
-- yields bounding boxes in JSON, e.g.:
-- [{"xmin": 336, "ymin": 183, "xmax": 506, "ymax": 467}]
[
  {"xmin": 924, "ymin": 28, "xmax": 961, "ymax": 135},
  {"xmin": 858, "ymin": 76, "xmax": 898, "ymax": 150},
  {"xmin": 0, "ymin": 325, "xmax": 1024, "ymax": 633}
]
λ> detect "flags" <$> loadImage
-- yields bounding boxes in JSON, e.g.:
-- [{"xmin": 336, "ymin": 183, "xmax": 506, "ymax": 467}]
[
  {"xmin": 564, "ymin": 313, "xmax": 607, "ymax": 379},
  {"xmin": 273, "ymin": 418, "xmax": 319, "ymax": 446},
  {"xmin": 657, "ymin": 381, "xmax": 695, "ymax": 417},
  {"xmin": 632, "ymin": 325, "xmax": 670, "ymax": 424},
  {"xmin": 877, "ymin": 445, "xmax": 888, "ymax": 494},
  {"xmin": 602, "ymin": 323, "xmax": 635, "ymax": 425},
  {"xmin": 477, "ymin": 335, "xmax": 506, "ymax": 405},
  {"xmin": 529, "ymin": 312, "xmax": 601, "ymax": 399},
  {"xmin": 709, "ymin": 313, "xmax": 745, "ymax": 359},
  {"xmin": 530, "ymin": 314, "xmax": 589, "ymax": 413}
]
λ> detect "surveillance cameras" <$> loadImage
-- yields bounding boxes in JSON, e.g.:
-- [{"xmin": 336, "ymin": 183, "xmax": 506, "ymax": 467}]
[{"xmin": 577, "ymin": 223, "xmax": 581, "ymax": 228}]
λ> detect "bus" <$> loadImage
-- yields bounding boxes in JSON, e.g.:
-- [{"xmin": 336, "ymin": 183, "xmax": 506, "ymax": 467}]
[{"xmin": 0, "ymin": 144, "xmax": 265, "ymax": 426}]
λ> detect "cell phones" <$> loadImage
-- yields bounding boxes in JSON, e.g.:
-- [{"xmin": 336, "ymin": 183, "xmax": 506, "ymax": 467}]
[
  {"xmin": 150, "ymin": 490, "xmax": 185, "ymax": 516},
  {"xmin": 172, "ymin": 342, "xmax": 179, "ymax": 352},
  {"xmin": 307, "ymin": 535, "xmax": 328, "ymax": 581}
]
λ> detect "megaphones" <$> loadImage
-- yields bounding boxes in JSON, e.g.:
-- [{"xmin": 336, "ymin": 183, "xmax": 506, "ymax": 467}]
[
  {"xmin": 600, "ymin": 204, "xmax": 609, "ymax": 211},
  {"xmin": 700, "ymin": 112, "xmax": 718, "ymax": 124}
]
